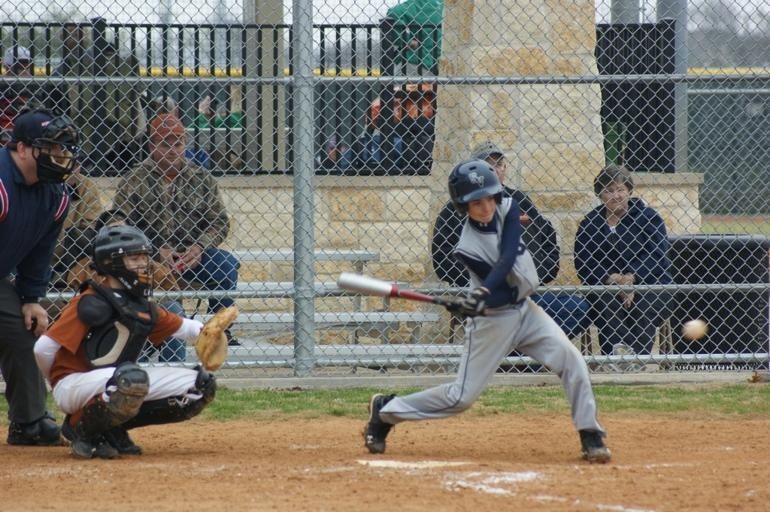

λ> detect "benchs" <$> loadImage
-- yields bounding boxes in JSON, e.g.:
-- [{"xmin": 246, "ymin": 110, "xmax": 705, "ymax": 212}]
[{"xmin": 178, "ymin": 248, "xmax": 466, "ymax": 366}]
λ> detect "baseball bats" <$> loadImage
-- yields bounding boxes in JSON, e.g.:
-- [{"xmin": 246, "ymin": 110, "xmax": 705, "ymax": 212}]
[{"xmin": 338, "ymin": 273, "xmax": 486, "ymax": 317}]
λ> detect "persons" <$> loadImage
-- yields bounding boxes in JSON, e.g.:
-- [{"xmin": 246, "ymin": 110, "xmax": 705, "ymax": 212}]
[
  {"xmin": 33, "ymin": 225, "xmax": 216, "ymax": 461},
  {"xmin": 46, "ymin": 164, "xmax": 104, "ymax": 292},
  {"xmin": 575, "ymin": 164, "xmax": 674, "ymax": 372},
  {"xmin": 432, "ymin": 141, "xmax": 590, "ymax": 372},
  {"xmin": 67, "ymin": 211, "xmax": 185, "ymax": 362},
  {"xmin": 113, "ymin": 113, "xmax": 242, "ymax": 345},
  {"xmin": 0, "ymin": 44, "xmax": 36, "ymax": 147},
  {"xmin": 141, "ymin": 94, "xmax": 211, "ymax": 177},
  {"xmin": 364, "ymin": 160, "xmax": 612, "ymax": 462},
  {"xmin": 0, "ymin": 108, "xmax": 84, "ymax": 447}
]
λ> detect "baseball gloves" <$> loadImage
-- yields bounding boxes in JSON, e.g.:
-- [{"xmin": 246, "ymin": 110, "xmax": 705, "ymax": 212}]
[{"xmin": 197, "ymin": 305, "xmax": 239, "ymax": 369}]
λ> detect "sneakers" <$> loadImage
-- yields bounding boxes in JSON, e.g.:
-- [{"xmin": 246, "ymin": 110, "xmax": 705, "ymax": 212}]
[
  {"xmin": 6, "ymin": 418, "xmax": 66, "ymax": 446},
  {"xmin": 106, "ymin": 426, "xmax": 142, "ymax": 454},
  {"xmin": 580, "ymin": 427, "xmax": 611, "ymax": 464},
  {"xmin": 59, "ymin": 414, "xmax": 118, "ymax": 460},
  {"xmin": 363, "ymin": 392, "xmax": 396, "ymax": 454},
  {"xmin": 225, "ymin": 327, "xmax": 241, "ymax": 346}
]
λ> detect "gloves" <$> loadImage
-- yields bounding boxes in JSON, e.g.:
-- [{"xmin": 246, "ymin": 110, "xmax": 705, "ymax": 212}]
[{"xmin": 447, "ymin": 286, "xmax": 491, "ymax": 319}]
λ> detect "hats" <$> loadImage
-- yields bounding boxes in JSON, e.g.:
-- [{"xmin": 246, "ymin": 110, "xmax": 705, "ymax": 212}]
[
  {"xmin": 3, "ymin": 46, "xmax": 34, "ymax": 67},
  {"xmin": 470, "ymin": 141, "xmax": 507, "ymax": 160},
  {"xmin": 12, "ymin": 111, "xmax": 80, "ymax": 148},
  {"xmin": 147, "ymin": 113, "xmax": 186, "ymax": 141}
]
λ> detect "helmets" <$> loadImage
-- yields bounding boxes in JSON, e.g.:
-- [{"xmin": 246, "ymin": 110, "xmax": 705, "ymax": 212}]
[
  {"xmin": 448, "ymin": 158, "xmax": 503, "ymax": 214},
  {"xmin": 92, "ymin": 225, "xmax": 156, "ymax": 299}
]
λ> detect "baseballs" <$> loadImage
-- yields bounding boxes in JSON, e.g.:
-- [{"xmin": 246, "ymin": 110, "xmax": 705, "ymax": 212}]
[{"xmin": 681, "ymin": 318, "xmax": 708, "ymax": 339}]
